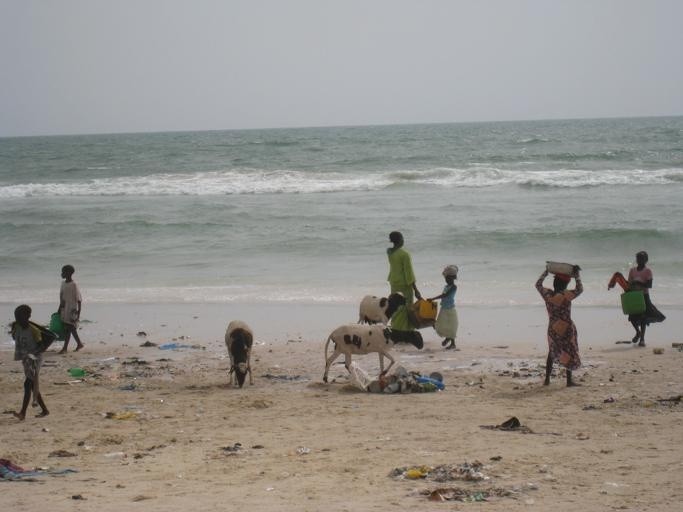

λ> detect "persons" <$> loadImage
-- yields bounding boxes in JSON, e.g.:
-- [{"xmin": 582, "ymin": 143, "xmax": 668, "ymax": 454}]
[
  {"xmin": 56, "ymin": 264, "xmax": 84, "ymax": 355},
  {"xmin": 386, "ymin": 231, "xmax": 421, "ymax": 331},
  {"xmin": 10, "ymin": 306, "xmax": 49, "ymax": 421},
  {"xmin": 426, "ymin": 264, "xmax": 459, "ymax": 350},
  {"xmin": 535, "ymin": 265, "xmax": 583, "ymax": 387},
  {"xmin": 614, "ymin": 250, "xmax": 666, "ymax": 346}
]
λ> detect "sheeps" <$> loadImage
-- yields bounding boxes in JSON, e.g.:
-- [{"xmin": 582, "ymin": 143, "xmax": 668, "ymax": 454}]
[
  {"xmin": 322, "ymin": 324, "xmax": 425, "ymax": 382},
  {"xmin": 355, "ymin": 291, "xmax": 407, "ymax": 326}
]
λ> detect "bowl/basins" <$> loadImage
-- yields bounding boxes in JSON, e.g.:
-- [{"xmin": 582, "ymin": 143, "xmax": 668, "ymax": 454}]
[{"xmin": 546, "ymin": 259, "xmax": 576, "ymax": 277}]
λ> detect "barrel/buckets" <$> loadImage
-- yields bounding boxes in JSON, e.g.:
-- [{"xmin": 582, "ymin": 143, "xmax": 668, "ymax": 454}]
[
  {"xmin": 49, "ymin": 313, "xmax": 66, "ymax": 341},
  {"xmin": 621, "ymin": 291, "xmax": 646, "ymax": 314},
  {"xmin": 414, "ymin": 298, "xmax": 437, "ymax": 319}
]
